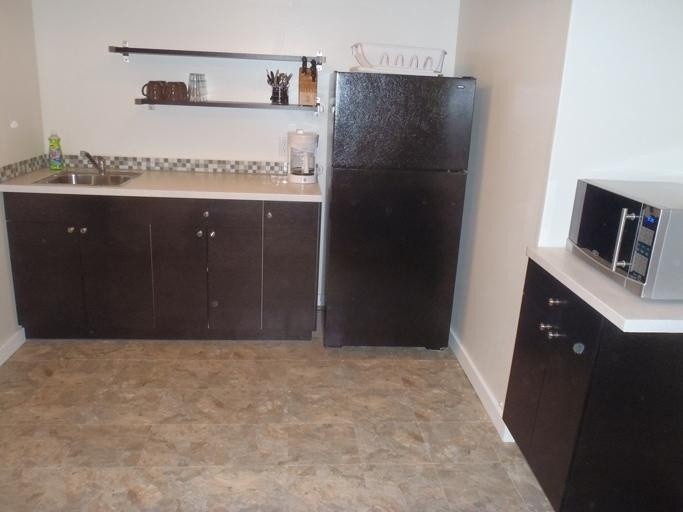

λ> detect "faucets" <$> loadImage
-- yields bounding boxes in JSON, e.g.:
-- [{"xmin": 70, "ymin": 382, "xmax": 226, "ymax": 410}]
[{"xmin": 79, "ymin": 150, "xmax": 106, "ymax": 176}]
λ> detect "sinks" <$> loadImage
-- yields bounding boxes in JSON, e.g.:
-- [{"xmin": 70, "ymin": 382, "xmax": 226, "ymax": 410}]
[{"xmin": 31, "ymin": 170, "xmax": 143, "ymax": 187}]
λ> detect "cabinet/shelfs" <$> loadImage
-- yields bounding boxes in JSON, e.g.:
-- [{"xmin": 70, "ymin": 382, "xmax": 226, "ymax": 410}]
[
  {"xmin": 147, "ymin": 200, "xmax": 262, "ymax": 340},
  {"xmin": 106, "ymin": 42, "xmax": 327, "ymax": 112},
  {"xmin": 501, "ymin": 257, "xmax": 682, "ymax": 511},
  {"xmin": 262, "ymin": 202, "xmax": 321, "ymax": 341},
  {"xmin": 2, "ymin": 192, "xmax": 154, "ymax": 341}
]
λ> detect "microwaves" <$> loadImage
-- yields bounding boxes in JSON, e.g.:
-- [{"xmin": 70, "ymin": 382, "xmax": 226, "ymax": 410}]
[{"xmin": 563, "ymin": 178, "xmax": 682, "ymax": 302}]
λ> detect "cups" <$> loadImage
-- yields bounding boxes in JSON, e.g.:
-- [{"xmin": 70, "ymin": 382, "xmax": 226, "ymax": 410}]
[
  {"xmin": 139, "ymin": 80, "xmax": 187, "ymax": 102},
  {"xmin": 188, "ymin": 74, "xmax": 208, "ymax": 105},
  {"xmin": 271, "ymin": 87, "xmax": 288, "ymax": 105}
]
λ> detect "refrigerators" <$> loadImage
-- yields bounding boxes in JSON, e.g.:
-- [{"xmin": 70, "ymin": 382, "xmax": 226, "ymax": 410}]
[{"xmin": 320, "ymin": 72, "xmax": 478, "ymax": 353}]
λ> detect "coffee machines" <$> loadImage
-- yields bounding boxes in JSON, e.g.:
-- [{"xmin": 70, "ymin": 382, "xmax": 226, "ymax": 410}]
[{"xmin": 286, "ymin": 129, "xmax": 319, "ymax": 184}]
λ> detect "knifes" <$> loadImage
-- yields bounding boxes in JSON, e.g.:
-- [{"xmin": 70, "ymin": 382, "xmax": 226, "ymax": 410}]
[{"xmin": 300, "ymin": 57, "xmax": 316, "ymax": 81}]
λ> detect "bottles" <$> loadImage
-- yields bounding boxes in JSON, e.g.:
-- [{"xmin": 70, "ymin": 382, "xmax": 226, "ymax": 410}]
[{"xmin": 47, "ymin": 129, "xmax": 63, "ymax": 171}]
